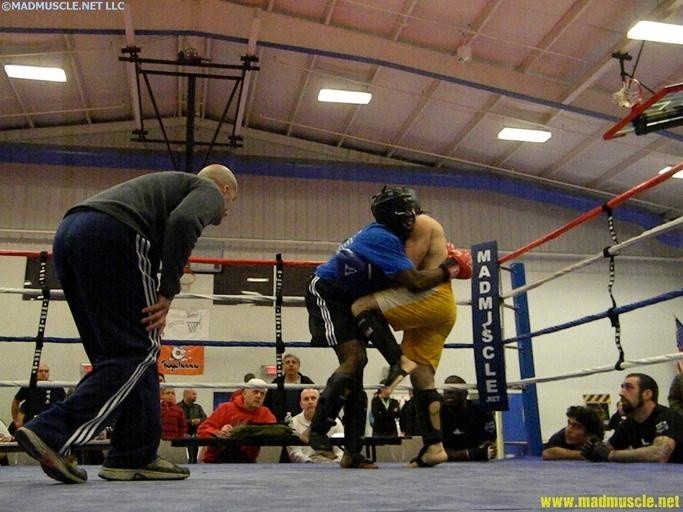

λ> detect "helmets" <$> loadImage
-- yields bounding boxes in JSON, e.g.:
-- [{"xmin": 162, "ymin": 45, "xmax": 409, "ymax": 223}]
[{"xmin": 371, "ymin": 186, "xmax": 417, "ymax": 233}]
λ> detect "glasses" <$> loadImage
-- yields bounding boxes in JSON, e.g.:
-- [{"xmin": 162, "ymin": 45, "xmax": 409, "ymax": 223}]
[{"xmin": 163, "ymin": 392, "xmax": 175, "ymax": 395}]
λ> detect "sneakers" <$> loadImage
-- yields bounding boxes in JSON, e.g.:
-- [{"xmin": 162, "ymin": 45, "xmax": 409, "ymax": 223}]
[
  {"xmin": 14, "ymin": 426, "xmax": 88, "ymax": 483},
  {"xmin": 98, "ymin": 452, "xmax": 190, "ymax": 480}
]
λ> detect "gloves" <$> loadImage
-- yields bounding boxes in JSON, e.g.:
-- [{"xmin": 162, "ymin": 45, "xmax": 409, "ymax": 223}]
[
  {"xmin": 581, "ymin": 435, "xmax": 610, "ymax": 463},
  {"xmin": 439, "ymin": 242, "xmax": 474, "ymax": 280},
  {"xmin": 468, "ymin": 442, "xmax": 497, "ymax": 460}
]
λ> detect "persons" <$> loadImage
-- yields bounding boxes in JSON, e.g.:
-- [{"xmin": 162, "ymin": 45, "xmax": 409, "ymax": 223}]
[
  {"xmin": 286, "ymin": 387, "xmax": 345, "ymax": 464},
  {"xmin": 196, "ymin": 378, "xmax": 276, "ymax": 464},
  {"xmin": 159, "ymin": 386, "xmax": 187, "ymax": 441},
  {"xmin": 351, "ymin": 188, "xmax": 458, "ymax": 468},
  {"xmin": 582, "ymin": 372, "xmax": 683, "ymax": 462},
  {"xmin": 17, "ymin": 164, "xmax": 239, "ymax": 482},
  {"xmin": 400, "ymin": 388, "xmax": 419, "ymax": 436},
  {"xmin": 541, "ymin": 405, "xmax": 605, "ymax": 460},
  {"xmin": 368, "ymin": 378, "xmax": 399, "ymax": 435},
  {"xmin": 263, "ymin": 354, "xmax": 314, "ymax": 422},
  {"xmin": 441, "ymin": 375, "xmax": 497, "ymax": 462},
  {"xmin": 177, "ymin": 387, "xmax": 207, "ymax": 436},
  {"xmin": 305, "ymin": 185, "xmax": 473, "ymax": 468},
  {"xmin": 11, "ymin": 363, "xmax": 66, "ymax": 439}
]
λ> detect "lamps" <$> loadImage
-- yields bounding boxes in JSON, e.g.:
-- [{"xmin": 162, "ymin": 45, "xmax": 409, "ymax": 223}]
[
  {"xmin": 4, "ymin": 64, "xmax": 68, "ymax": 83},
  {"xmin": 496, "ymin": 127, "xmax": 553, "ymax": 144},
  {"xmin": 626, "ymin": 21, "xmax": 683, "ymax": 45},
  {"xmin": 658, "ymin": 165, "xmax": 683, "ymax": 179},
  {"xmin": 317, "ymin": 89, "xmax": 372, "ymax": 105}
]
